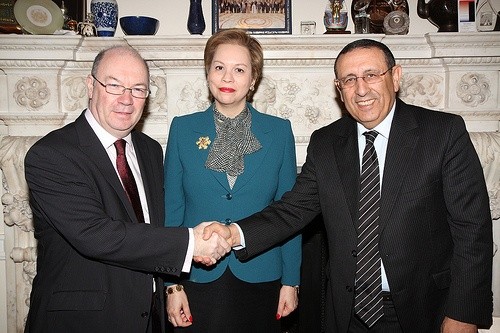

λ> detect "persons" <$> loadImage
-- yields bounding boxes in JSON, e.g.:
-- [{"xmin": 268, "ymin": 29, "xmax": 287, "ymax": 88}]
[
  {"xmin": 163, "ymin": 30, "xmax": 302, "ymax": 333},
  {"xmin": 24, "ymin": 46, "xmax": 231, "ymax": 333},
  {"xmin": 195, "ymin": 39, "xmax": 494, "ymax": 333}
]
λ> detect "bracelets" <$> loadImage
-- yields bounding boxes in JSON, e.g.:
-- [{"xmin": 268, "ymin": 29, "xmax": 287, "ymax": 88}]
[
  {"xmin": 165, "ymin": 285, "xmax": 184, "ymax": 294},
  {"xmin": 292, "ymin": 286, "xmax": 299, "ymax": 290}
]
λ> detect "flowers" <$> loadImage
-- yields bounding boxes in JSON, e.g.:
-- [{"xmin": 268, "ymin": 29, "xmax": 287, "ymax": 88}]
[{"xmin": 197, "ymin": 136, "xmax": 211, "ymax": 150}]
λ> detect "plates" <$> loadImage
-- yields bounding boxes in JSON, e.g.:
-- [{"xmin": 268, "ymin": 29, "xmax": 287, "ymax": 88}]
[{"xmin": 13, "ymin": 0, "xmax": 64, "ymax": 33}]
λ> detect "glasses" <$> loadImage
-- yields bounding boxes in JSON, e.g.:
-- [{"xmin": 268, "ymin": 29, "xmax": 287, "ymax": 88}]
[
  {"xmin": 337, "ymin": 65, "xmax": 395, "ymax": 88},
  {"xmin": 91, "ymin": 74, "xmax": 152, "ymax": 100}
]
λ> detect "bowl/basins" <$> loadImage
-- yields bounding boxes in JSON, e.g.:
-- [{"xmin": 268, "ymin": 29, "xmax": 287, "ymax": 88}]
[{"xmin": 118, "ymin": 16, "xmax": 159, "ymax": 36}]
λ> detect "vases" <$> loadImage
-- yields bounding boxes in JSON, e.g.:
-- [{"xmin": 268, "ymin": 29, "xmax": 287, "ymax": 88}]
[
  {"xmin": 91, "ymin": 0, "xmax": 118, "ymax": 37},
  {"xmin": 187, "ymin": 0, "xmax": 205, "ymax": 35}
]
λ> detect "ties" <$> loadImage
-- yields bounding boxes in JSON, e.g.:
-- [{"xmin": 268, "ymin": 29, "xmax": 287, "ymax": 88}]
[
  {"xmin": 114, "ymin": 139, "xmax": 145, "ymax": 224},
  {"xmin": 353, "ymin": 130, "xmax": 385, "ymax": 327}
]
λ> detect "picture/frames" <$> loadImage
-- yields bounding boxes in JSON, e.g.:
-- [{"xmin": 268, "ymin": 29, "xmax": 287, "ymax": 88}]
[{"xmin": 212, "ymin": 0, "xmax": 292, "ymax": 35}]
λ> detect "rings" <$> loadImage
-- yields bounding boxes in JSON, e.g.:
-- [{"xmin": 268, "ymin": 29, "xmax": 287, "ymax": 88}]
[{"xmin": 168, "ymin": 319, "xmax": 171, "ymax": 322}]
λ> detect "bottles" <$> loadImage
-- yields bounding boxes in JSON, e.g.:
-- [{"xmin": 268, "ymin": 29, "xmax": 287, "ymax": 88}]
[
  {"xmin": 187, "ymin": 1, "xmax": 206, "ymax": 35},
  {"xmin": 323, "ymin": 0, "xmax": 348, "ymax": 31}
]
